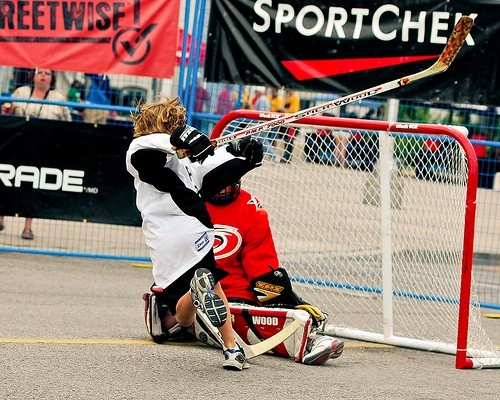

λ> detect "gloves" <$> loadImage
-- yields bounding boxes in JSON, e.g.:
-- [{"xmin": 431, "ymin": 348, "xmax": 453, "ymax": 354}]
[
  {"xmin": 226, "ymin": 134, "xmax": 263, "ymax": 164},
  {"xmin": 170, "ymin": 125, "xmax": 214, "ymax": 164}
]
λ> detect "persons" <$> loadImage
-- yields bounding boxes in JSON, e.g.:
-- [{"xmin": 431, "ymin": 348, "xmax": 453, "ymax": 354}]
[
  {"xmin": 126, "ymin": 91, "xmax": 252, "ymax": 372},
  {"xmin": 182, "ymin": 77, "xmax": 301, "ymax": 165},
  {"xmin": 67, "ymin": 79, "xmax": 85, "ymax": 121},
  {"xmin": 205, "ymin": 177, "xmax": 345, "ymax": 367},
  {"xmin": 0, "ymin": 68, "xmax": 71, "ymax": 240}
]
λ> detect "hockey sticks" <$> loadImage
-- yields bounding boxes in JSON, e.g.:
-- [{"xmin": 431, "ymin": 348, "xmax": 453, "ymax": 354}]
[
  {"xmin": 195, "ymin": 307, "xmax": 301, "ymax": 362},
  {"xmin": 176, "ymin": 16, "xmax": 475, "ymax": 158}
]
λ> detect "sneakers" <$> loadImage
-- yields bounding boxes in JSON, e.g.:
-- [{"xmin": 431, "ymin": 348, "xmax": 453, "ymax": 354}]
[
  {"xmin": 191, "ymin": 268, "xmax": 227, "ymax": 328},
  {"xmin": 301, "ymin": 336, "xmax": 344, "ymax": 366},
  {"xmin": 222, "ymin": 343, "xmax": 249, "ymax": 371}
]
